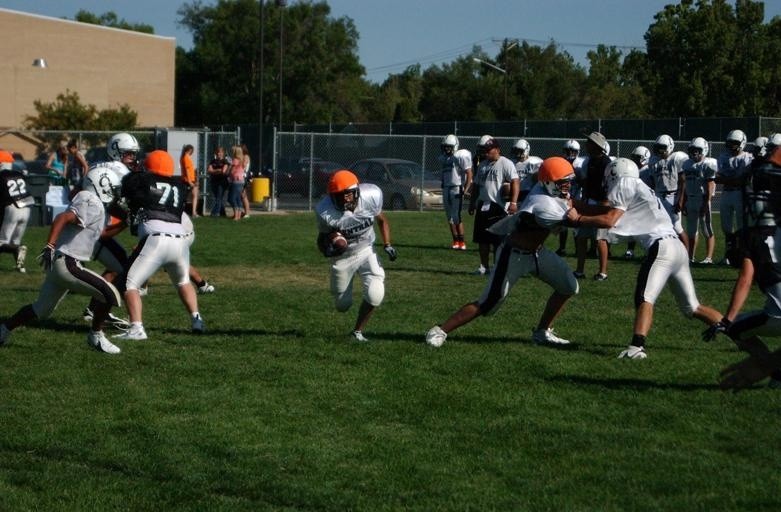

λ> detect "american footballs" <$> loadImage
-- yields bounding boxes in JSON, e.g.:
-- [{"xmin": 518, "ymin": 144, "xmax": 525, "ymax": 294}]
[{"xmin": 329, "ymin": 231, "xmax": 348, "ymax": 249}]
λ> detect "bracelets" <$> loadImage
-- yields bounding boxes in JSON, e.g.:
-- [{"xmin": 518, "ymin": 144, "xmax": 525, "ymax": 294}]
[{"xmin": 578, "ymin": 215, "xmax": 584, "ymax": 223}]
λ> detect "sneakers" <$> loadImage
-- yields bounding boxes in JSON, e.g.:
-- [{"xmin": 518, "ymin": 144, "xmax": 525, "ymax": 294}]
[
  {"xmin": 450, "ymin": 240, "xmax": 468, "ymax": 251},
  {"xmin": 530, "ymin": 326, "xmax": 571, "ymax": 347},
  {"xmin": 348, "ymin": 328, "xmax": 369, "ymax": 343},
  {"xmin": 474, "ymin": 263, "xmax": 489, "ymax": 277},
  {"xmin": 0, "ymin": 315, "xmax": 13, "ymax": 348},
  {"xmin": 189, "ymin": 314, "xmax": 206, "ymax": 334},
  {"xmin": 87, "ymin": 332, "xmax": 121, "ymax": 355},
  {"xmin": 81, "ymin": 307, "xmax": 122, "ymax": 323},
  {"xmin": 197, "ymin": 280, "xmax": 216, "ymax": 296},
  {"xmin": 553, "ymin": 247, "xmax": 737, "ymax": 282},
  {"xmin": 616, "ymin": 343, "xmax": 648, "ymax": 361},
  {"xmin": 422, "ymin": 323, "xmax": 448, "ymax": 349},
  {"xmin": 11, "ymin": 243, "xmax": 29, "ymax": 274},
  {"xmin": 111, "ymin": 324, "xmax": 149, "ymax": 340}
]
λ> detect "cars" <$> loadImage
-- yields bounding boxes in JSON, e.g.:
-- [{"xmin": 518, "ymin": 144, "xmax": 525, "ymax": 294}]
[
  {"xmin": 22, "ymin": 145, "xmax": 144, "ymax": 184},
  {"xmin": 198, "ymin": 154, "xmax": 445, "ymax": 211}
]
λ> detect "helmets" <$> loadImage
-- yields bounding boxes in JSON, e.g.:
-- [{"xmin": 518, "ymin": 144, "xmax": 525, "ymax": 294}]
[
  {"xmin": 513, "ymin": 140, "xmax": 531, "ymax": 157},
  {"xmin": 755, "ymin": 133, "xmax": 781, "ymax": 146},
  {"xmin": 604, "ymin": 157, "xmax": 640, "ymax": 191},
  {"xmin": 725, "ymin": 129, "xmax": 747, "ymax": 150},
  {"xmin": 0, "ymin": 150, "xmax": 16, "ymax": 162},
  {"xmin": 538, "ymin": 156, "xmax": 576, "ymax": 195},
  {"xmin": 688, "ymin": 137, "xmax": 710, "ymax": 157},
  {"xmin": 440, "ymin": 134, "xmax": 460, "ymax": 154},
  {"xmin": 477, "ymin": 134, "xmax": 494, "ymax": 147},
  {"xmin": 632, "ymin": 145, "xmax": 651, "ymax": 165},
  {"xmin": 562, "ymin": 139, "xmax": 580, "ymax": 152},
  {"xmin": 81, "ymin": 133, "xmax": 176, "ymax": 203},
  {"xmin": 328, "ymin": 168, "xmax": 360, "ymax": 212},
  {"xmin": 654, "ymin": 134, "xmax": 674, "ymax": 154}
]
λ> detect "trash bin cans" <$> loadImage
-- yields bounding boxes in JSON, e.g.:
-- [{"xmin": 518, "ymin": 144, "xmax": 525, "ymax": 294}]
[
  {"xmin": 250, "ymin": 167, "xmax": 273, "ymax": 204},
  {"xmin": 26, "ymin": 174, "xmax": 52, "ymax": 227}
]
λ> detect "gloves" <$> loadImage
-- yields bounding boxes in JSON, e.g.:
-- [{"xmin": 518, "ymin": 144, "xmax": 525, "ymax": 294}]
[
  {"xmin": 34, "ymin": 241, "xmax": 57, "ymax": 273},
  {"xmin": 383, "ymin": 241, "xmax": 398, "ymax": 263},
  {"xmin": 700, "ymin": 320, "xmax": 732, "ymax": 343}
]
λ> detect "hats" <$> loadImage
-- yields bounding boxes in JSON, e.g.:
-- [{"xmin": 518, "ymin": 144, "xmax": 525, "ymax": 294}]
[
  {"xmin": 480, "ymin": 138, "xmax": 500, "ymax": 153},
  {"xmin": 581, "ymin": 131, "xmax": 610, "ymax": 153}
]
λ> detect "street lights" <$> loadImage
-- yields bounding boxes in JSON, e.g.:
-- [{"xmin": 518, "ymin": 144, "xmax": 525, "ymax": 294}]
[{"xmin": 473, "ymin": 56, "xmax": 510, "ymax": 122}]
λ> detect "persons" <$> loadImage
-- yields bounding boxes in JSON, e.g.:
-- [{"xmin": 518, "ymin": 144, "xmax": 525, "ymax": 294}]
[
  {"xmin": 439, "ymin": 129, "xmax": 780, "ymax": 280},
  {"xmin": 427, "ymin": 157, "xmax": 614, "ymax": 348},
  {"xmin": 2, "ymin": 168, "xmax": 122, "ymax": 352},
  {"xmin": 31, "ymin": 137, "xmax": 88, "ymax": 200},
  {"xmin": 109, "ymin": 151, "xmax": 214, "ymax": 342},
  {"xmin": 565, "ymin": 157, "xmax": 729, "ymax": 359},
  {"xmin": 180, "ymin": 144, "xmax": 251, "ymax": 219},
  {"xmin": 316, "ymin": 169, "xmax": 398, "ymax": 342},
  {"xmin": 698, "ymin": 187, "xmax": 781, "ymax": 395},
  {"xmin": 1, "ymin": 152, "xmax": 33, "ymax": 272},
  {"xmin": 70, "ymin": 133, "xmax": 142, "ymax": 328}
]
